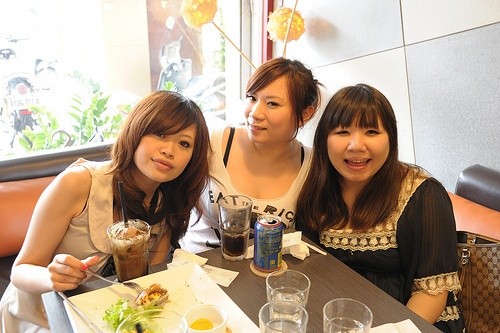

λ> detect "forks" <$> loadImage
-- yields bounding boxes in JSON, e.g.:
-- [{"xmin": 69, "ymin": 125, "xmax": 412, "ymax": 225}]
[{"xmin": 85, "ymin": 267, "xmax": 144, "ymax": 296}]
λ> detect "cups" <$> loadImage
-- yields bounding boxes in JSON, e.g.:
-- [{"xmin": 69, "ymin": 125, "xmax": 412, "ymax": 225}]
[
  {"xmin": 107, "ymin": 219, "xmax": 151, "ymax": 283},
  {"xmin": 322, "ymin": 297, "xmax": 373, "ymax": 333},
  {"xmin": 266, "ymin": 269, "xmax": 311, "ymax": 325},
  {"xmin": 182, "ymin": 303, "xmax": 228, "ymax": 333},
  {"xmin": 218, "ymin": 195, "xmax": 254, "ymax": 262},
  {"xmin": 115, "ymin": 307, "xmax": 189, "ymax": 333},
  {"xmin": 258, "ymin": 299, "xmax": 309, "ymax": 333}
]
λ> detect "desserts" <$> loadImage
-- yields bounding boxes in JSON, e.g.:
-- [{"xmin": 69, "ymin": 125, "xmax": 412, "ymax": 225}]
[{"xmin": 136, "ymin": 284, "xmax": 169, "ymax": 307}]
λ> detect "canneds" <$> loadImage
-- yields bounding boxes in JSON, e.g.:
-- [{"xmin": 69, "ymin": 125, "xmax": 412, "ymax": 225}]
[{"xmin": 254, "ymin": 214, "xmax": 283, "ymax": 273}]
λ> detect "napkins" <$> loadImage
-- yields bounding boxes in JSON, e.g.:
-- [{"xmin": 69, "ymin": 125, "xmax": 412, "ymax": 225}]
[
  {"xmin": 168, "ymin": 247, "xmax": 208, "ymax": 270},
  {"xmin": 370, "ymin": 317, "xmax": 422, "ymax": 333}
]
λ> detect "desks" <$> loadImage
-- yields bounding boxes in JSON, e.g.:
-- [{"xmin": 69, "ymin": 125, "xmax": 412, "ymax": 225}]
[{"xmin": 41, "ymin": 228, "xmax": 445, "ymax": 333}]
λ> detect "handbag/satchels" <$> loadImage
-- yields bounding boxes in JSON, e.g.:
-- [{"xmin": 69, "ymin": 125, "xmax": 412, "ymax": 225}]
[{"xmin": 453, "ymin": 230, "xmax": 500, "ymax": 333}]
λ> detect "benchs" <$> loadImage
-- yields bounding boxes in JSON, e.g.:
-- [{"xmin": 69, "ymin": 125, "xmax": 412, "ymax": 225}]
[
  {"xmin": 447, "ymin": 164, "xmax": 500, "ymax": 241},
  {"xmin": 0, "ymin": 141, "xmax": 117, "ymax": 302}
]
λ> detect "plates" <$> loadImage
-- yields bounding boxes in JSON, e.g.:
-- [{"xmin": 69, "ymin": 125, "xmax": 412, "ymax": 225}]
[{"xmin": 63, "ymin": 262, "xmax": 262, "ymax": 333}]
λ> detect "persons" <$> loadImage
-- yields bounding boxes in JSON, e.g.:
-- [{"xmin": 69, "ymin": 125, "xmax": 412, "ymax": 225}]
[
  {"xmin": 175, "ymin": 58, "xmax": 326, "ymax": 255},
  {"xmin": 294, "ymin": 82, "xmax": 466, "ymax": 333},
  {"xmin": 0, "ymin": 89, "xmax": 213, "ymax": 333}
]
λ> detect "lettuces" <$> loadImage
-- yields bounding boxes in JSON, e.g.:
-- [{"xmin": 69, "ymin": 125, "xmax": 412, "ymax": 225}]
[{"xmin": 103, "ymin": 299, "xmax": 165, "ymax": 333}]
